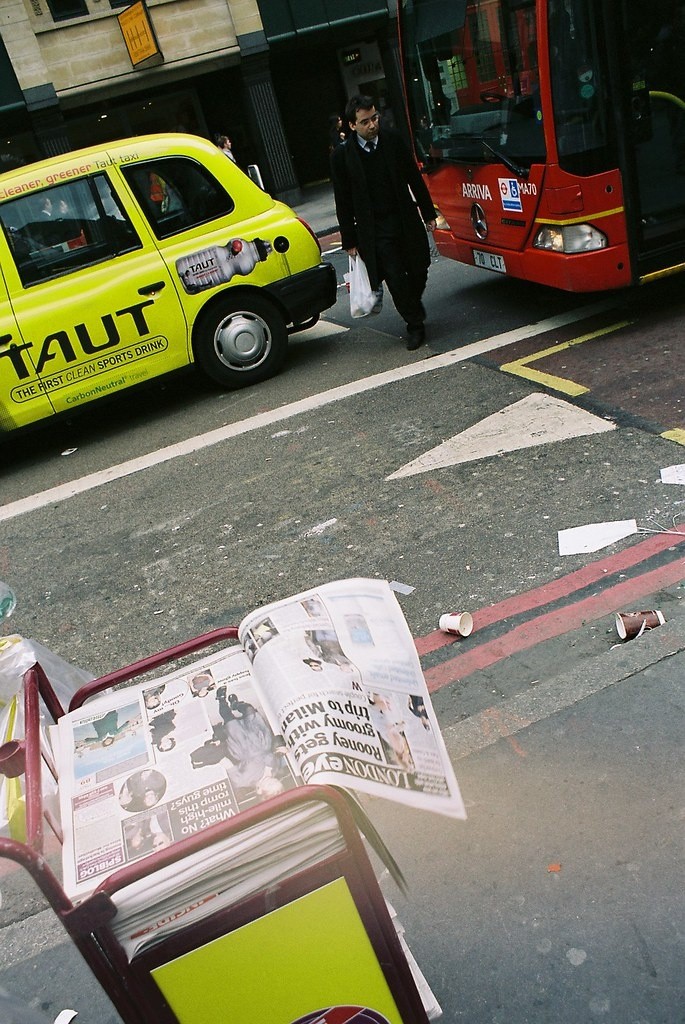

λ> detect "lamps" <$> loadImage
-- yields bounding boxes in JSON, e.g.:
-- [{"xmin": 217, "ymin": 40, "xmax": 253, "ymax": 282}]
[
  {"xmin": 3, "ymin": 136, "xmax": 15, "ymax": 146},
  {"xmin": 136, "ymin": 97, "xmax": 152, "ymax": 114},
  {"xmin": 95, "ymin": 111, "xmax": 111, "ymax": 129}
]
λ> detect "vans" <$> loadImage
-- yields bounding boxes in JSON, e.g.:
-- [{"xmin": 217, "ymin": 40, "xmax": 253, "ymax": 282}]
[{"xmin": 1, "ymin": 132, "xmax": 337, "ymax": 454}]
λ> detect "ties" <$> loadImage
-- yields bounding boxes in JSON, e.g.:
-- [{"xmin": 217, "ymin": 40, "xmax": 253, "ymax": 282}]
[{"xmin": 366, "ymin": 141, "xmax": 375, "ymax": 154}]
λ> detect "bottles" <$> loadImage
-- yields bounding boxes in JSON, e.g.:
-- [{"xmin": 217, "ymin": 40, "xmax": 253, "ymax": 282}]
[{"xmin": 173, "ymin": 236, "xmax": 272, "ymax": 295}]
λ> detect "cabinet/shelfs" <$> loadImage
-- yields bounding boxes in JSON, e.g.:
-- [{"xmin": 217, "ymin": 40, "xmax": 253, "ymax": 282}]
[{"xmin": 0, "ymin": 626, "xmax": 430, "ymax": 1024}]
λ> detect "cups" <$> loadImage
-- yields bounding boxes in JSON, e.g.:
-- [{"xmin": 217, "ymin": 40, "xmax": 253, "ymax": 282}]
[
  {"xmin": 615, "ymin": 610, "xmax": 665, "ymax": 640},
  {"xmin": 439, "ymin": 612, "xmax": 473, "ymax": 637}
]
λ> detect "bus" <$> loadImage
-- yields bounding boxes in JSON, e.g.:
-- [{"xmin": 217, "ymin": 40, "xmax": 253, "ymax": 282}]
[{"xmin": 386, "ymin": 0, "xmax": 684, "ymax": 291}]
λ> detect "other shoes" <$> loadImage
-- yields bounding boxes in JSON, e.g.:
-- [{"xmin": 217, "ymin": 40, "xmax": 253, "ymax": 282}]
[
  {"xmin": 373, "ymin": 282, "xmax": 383, "ymax": 314},
  {"xmin": 406, "ymin": 321, "xmax": 425, "ymax": 351}
]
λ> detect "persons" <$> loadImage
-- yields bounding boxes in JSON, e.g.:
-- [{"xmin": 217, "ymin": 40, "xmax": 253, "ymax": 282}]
[
  {"xmin": 218, "ymin": 136, "xmax": 236, "ymax": 163},
  {"xmin": 330, "ymin": 94, "xmax": 438, "ymax": 352},
  {"xmin": 38, "ymin": 197, "xmax": 72, "ymax": 246},
  {"xmin": 150, "ymin": 172, "xmax": 168, "ymax": 202},
  {"xmin": 72, "ymin": 629, "xmax": 432, "ymax": 852},
  {"xmin": 330, "ymin": 115, "xmax": 345, "ymax": 150}
]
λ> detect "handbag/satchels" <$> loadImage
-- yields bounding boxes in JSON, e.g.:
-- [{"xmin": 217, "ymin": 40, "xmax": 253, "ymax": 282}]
[
  {"xmin": 0, "ymin": 633, "xmax": 115, "ymax": 879},
  {"xmin": 343, "ymin": 250, "xmax": 378, "ymax": 319}
]
